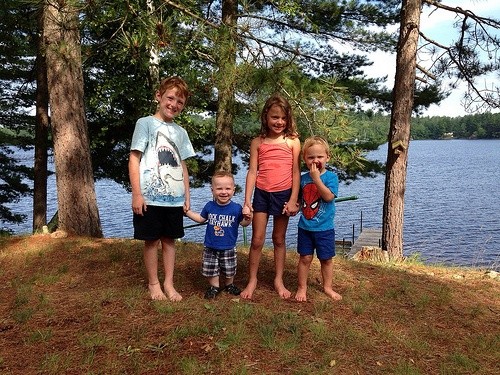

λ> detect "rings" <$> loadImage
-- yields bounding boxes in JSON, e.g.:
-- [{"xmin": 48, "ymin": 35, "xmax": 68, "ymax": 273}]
[{"xmin": 293, "ymin": 213, "xmax": 295, "ymax": 215}]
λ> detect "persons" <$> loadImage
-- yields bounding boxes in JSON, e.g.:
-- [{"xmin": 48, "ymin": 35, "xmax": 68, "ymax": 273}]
[
  {"xmin": 128, "ymin": 77, "xmax": 189, "ymax": 301},
  {"xmin": 183, "ymin": 171, "xmax": 253, "ymax": 300},
  {"xmin": 242, "ymin": 95, "xmax": 301, "ymax": 299},
  {"xmin": 284, "ymin": 135, "xmax": 342, "ymax": 302}
]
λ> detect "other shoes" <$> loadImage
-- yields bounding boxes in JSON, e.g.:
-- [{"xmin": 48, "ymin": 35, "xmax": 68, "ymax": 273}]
[
  {"xmin": 204, "ymin": 286, "xmax": 220, "ymax": 299},
  {"xmin": 225, "ymin": 284, "xmax": 241, "ymax": 295}
]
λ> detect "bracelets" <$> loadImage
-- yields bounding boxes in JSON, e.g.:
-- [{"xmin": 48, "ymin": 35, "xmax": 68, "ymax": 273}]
[{"xmin": 183, "ymin": 209, "xmax": 187, "ymax": 215}]
